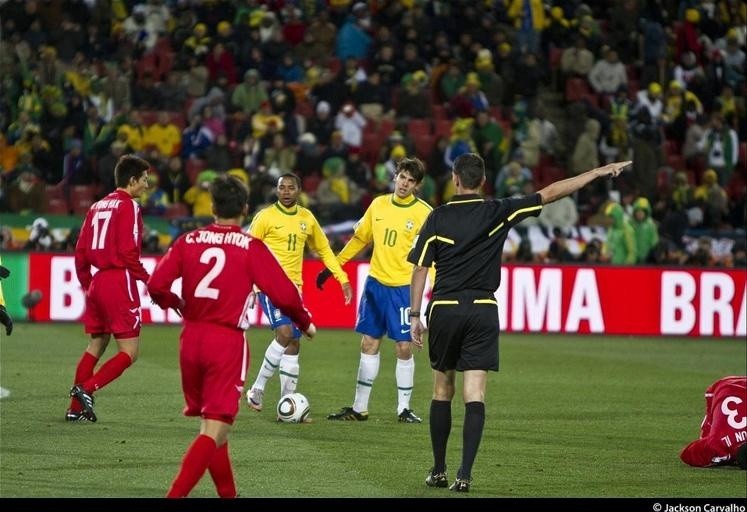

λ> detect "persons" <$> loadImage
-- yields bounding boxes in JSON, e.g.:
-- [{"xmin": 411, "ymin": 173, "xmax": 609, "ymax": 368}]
[
  {"xmin": 65, "ymin": 156, "xmax": 157, "ymax": 423},
  {"xmin": 315, "ymin": 158, "xmax": 437, "ymax": 424},
  {"xmin": 245, "ymin": 172, "xmax": 353, "ymax": 411},
  {"xmin": 61, "ymin": 226, "xmax": 79, "ymax": 255},
  {"xmin": 407, "ymin": 152, "xmax": 633, "ymax": 493},
  {"xmin": 501, "ymin": 198, "xmax": 747, "ymax": 269},
  {"xmin": 142, "ymin": 235, "xmax": 163, "ymax": 256},
  {"xmin": 0, "ymin": 257, "xmax": 13, "ymax": 401},
  {"xmin": 22, "ymin": 218, "xmax": 56, "ymax": 254},
  {"xmin": 3, "ymin": 1, "xmax": 63, "ymax": 219},
  {"xmin": 147, "ymin": 172, "xmax": 315, "ymax": 499},
  {"xmin": 680, "ymin": 375, "xmax": 747, "ymax": 471}
]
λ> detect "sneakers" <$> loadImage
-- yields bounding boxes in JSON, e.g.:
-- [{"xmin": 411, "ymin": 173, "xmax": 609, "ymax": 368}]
[
  {"xmin": 450, "ymin": 476, "xmax": 470, "ymax": 493},
  {"xmin": 69, "ymin": 384, "xmax": 97, "ymax": 422},
  {"xmin": 425, "ymin": 470, "xmax": 449, "ymax": 490},
  {"xmin": 326, "ymin": 407, "xmax": 370, "ymax": 422},
  {"xmin": 66, "ymin": 410, "xmax": 82, "ymax": 420},
  {"xmin": 1, "ymin": 387, "xmax": 11, "ymax": 400},
  {"xmin": 276, "ymin": 416, "xmax": 313, "ymax": 423},
  {"xmin": 398, "ymin": 408, "xmax": 422, "ymax": 425},
  {"xmin": 246, "ymin": 388, "xmax": 264, "ymax": 413}
]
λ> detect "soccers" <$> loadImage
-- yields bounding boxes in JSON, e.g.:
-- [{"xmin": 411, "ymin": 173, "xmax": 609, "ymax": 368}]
[{"xmin": 276, "ymin": 392, "xmax": 311, "ymax": 423}]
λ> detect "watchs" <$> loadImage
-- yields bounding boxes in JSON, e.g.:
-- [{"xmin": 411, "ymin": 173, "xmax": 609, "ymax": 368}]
[{"xmin": 408, "ymin": 310, "xmax": 420, "ymax": 317}]
